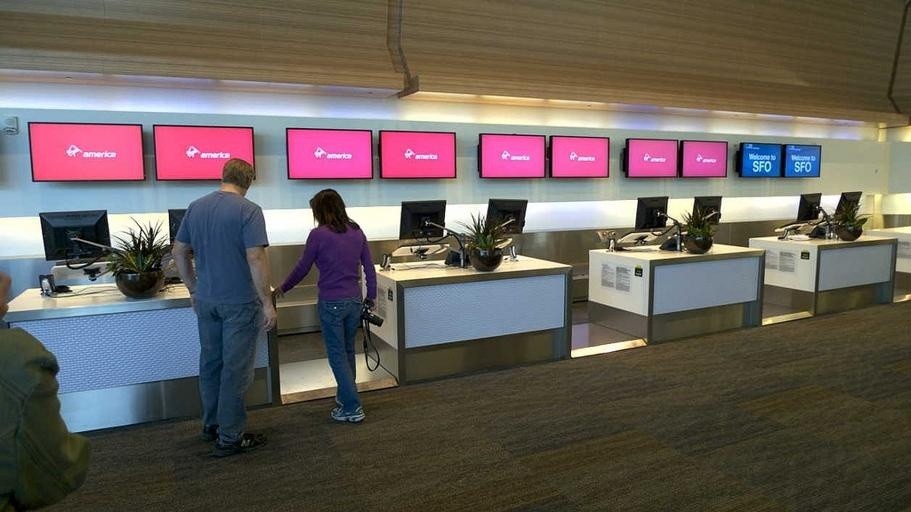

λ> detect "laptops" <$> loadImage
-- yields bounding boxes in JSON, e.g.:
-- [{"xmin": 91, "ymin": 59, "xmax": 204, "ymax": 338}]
[
  {"xmin": 38, "ymin": 208, "xmax": 116, "ymax": 292},
  {"xmin": 390, "ymin": 198, "xmax": 448, "ymax": 257},
  {"xmin": 461, "ymin": 198, "xmax": 530, "ymax": 252}
]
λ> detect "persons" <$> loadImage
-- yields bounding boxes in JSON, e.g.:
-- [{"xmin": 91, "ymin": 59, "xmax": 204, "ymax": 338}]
[
  {"xmin": 174, "ymin": 159, "xmax": 276, "ymax": 456},
  {"xmin": 273, "ymin": 190, "xmax": 377, "ymax": 423},
  {"xmin": 0, "ymin": 267, "xmax": 91, "ymax": 511}
]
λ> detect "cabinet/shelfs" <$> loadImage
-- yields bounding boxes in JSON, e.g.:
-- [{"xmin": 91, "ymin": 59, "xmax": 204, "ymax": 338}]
[
  {"xmin": 3, "ymin": 276, "xmax": 283, "ymax": 434},
  {"xmin": 587, "ymin": 243, "xmax": 768, "ymax": 345},
  {"xmin": 749, "ymin": 235, "xmax": 899, "ymax": 316},
  {"xmin": 361, "ymin": 254, "xmax": 574, "ymax": 387}
]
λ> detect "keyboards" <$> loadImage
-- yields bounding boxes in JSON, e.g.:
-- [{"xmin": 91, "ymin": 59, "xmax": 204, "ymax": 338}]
[
  {"xmin": 465, "ymin": 234, "xmax": 512, "ymax": 249},
  {"xmin": 392, "ymin": 244, "xmax": 450, "ymax": 257},
  {"xmin": 774, "ymin": 222, "xmax": 812, "ymax": 233},
  {"xmin": 617, "ymin": 232, "xmax": 662, "ymax": 244},
  {"xmin": 52, "ymin": 262, "xmax": 115, "ymax": 280}
]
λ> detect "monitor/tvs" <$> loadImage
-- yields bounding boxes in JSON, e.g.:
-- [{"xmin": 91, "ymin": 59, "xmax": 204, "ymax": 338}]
[
  {"xmin": 164, "ymin": 207, "xmax": 189, "ymax": 247},
  {"xmin": 735, "ymin": 142, "xmax": 782, "ymax": 178},
  {"xmin": 478, "ymin": 133, "xmax": 546, "ymax": 179},
  {"xmin": 635, "ymin": 196, "xmax": 668, "ymax": 229},
  {"xmin": 833, "ymin": 191, "xmax": 862, "ymax": 216},
  {"xmin": 797, "ymin": 193, "xmax": 822, "ymax": 220},
  {"xmin": 28, "ymin": 122, "xmax": 146, "ymax": 182},
  {"xmin": 547, "ymin": 136, "xmax": 610, "ymax": 178},
  {"xmin": 379, "ymin": 130, "xmax": 456, "ymax": 179},
  {"xmin": 784, "ymin": 144, "xmax": 821, "ymax": 178},
  {"xmin": 692, "ymin": 196, "xmax": 721, "ymax": 225},
  {"xmin": 623, "ymin": 138, "xmax": 678, "ymax": 178},
  {"xmin": 679, "ymin": 140, "xmax": 728, "ymax": 178},
  {"xmin": 286, "ymin": 128, "xmax": 374, "ymax": 180},
  {"xmin": 153, "ymin": 124, "xmax": 256, "ymax": 181}
]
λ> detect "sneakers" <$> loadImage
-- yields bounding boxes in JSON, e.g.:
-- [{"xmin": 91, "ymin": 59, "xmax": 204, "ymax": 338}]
[
  {"xmin": 198, "ymin": 422, "xmax": 220, "ymax": 442},
  {"xmin": 329, "ymin": 404, "xmax": 367, "ymax": 424},
  {"xmin": 213, "ymin": 431, "xmax": 272, "ymax": 459}
]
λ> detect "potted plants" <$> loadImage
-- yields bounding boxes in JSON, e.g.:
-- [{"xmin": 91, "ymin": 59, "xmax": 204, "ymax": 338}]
[
  {"xmin": 88, "ymin": 216, "xmax": 195, "ymax": 299},
  {"xmin": 450, "ymin": 211, "xmax": 516, "ymax": 271},
  {"xmin": 828, "ymin": 200, "xmax": 874, "ymax": 241},
  {"xmin": 673, "ymin": 206, "xmax": 722, "ymax": 254}
]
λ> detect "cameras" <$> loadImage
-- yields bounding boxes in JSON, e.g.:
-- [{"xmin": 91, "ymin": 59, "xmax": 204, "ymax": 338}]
[{"xmin": 361, "ymin": 307, "xmax": 384, "ymax": 327}]
